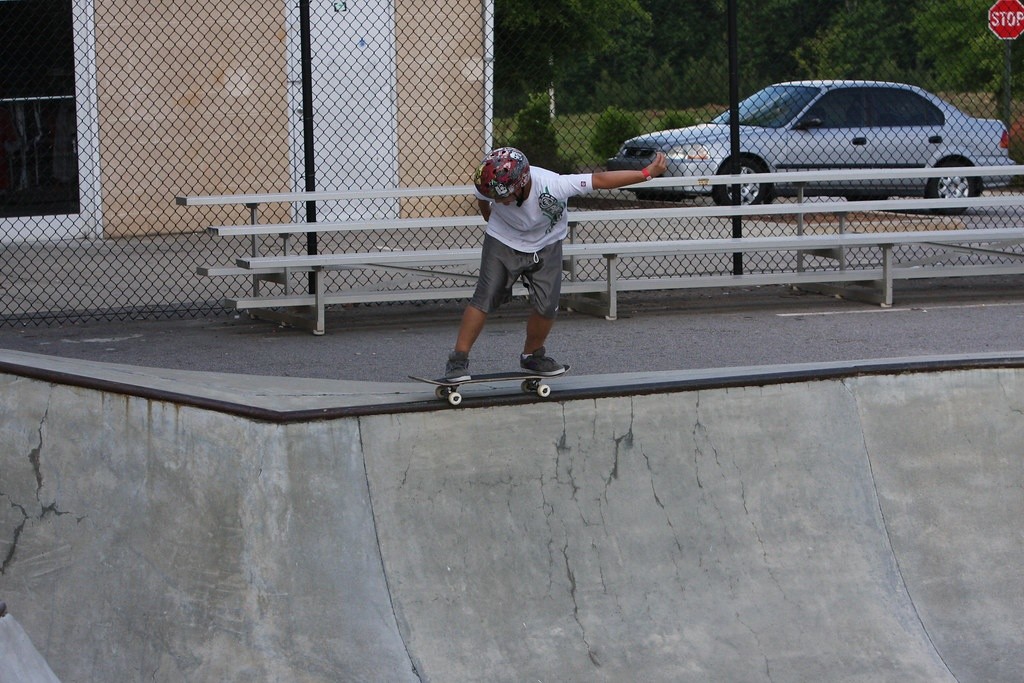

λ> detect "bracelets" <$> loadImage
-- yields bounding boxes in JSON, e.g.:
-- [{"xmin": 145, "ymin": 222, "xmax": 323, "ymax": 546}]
[{"xmin": 641, "ymin": 169, "xmax": 653, "ymax": 181}]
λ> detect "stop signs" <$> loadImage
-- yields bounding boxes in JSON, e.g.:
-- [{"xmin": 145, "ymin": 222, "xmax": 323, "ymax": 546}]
[{"xmin": 988, "ymin": 0, "xmax": 1024, "ymax": 41}]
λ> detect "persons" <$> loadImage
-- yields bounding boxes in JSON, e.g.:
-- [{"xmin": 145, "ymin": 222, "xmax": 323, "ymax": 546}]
[{"xmin": 446, "ymin": 144, "xmax": 668, "ymax": 382}]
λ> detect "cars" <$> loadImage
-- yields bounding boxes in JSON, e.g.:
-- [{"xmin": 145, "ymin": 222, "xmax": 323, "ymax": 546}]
[{"xmin": 605, "ymin": 79, "xmax": 1018, "ymax": 215}]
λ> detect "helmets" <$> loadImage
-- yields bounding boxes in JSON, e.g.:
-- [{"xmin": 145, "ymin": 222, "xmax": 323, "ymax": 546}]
[{"xmin": 474, "ymin": 147, "xmax": 530, "ymax": 199}]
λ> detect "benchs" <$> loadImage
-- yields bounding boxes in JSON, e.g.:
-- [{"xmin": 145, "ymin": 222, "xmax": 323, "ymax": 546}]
[{"xmin": 176, "ymin": 165, "xmax": 1024, "ymax": 335}]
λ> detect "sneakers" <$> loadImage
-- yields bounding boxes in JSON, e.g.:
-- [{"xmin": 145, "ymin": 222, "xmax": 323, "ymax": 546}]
[
  {"xmin": 445, "ymin": 351, "xmax": 471, "ymax": 382},
  {"xmin": 520, "ymin": 346, "xmax": 565, "ymax": 376}
]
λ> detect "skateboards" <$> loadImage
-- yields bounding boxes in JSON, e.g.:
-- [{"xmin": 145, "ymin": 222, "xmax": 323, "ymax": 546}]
[{"xmin": 407, "ymin": 364, "xmax": 573, "ymax": 406}]
